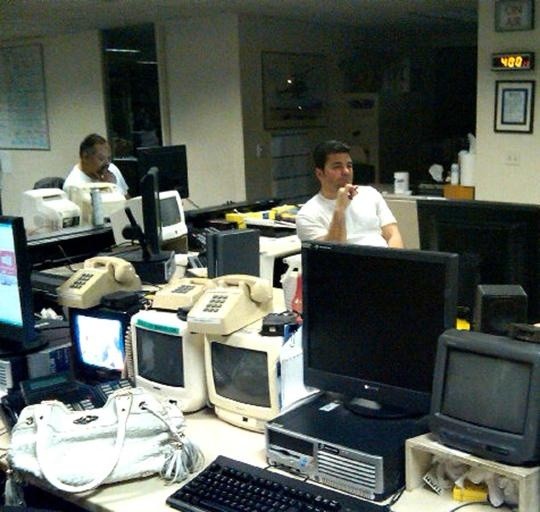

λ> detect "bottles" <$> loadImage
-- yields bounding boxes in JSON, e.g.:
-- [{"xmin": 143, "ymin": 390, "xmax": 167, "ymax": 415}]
[
  {"xmin": 90, "ymin": 189, "xmax": 106, "ymax": 227},
  {"xmin": 451, "ymin": 164, "xmax": 459, "ymax": 185}
]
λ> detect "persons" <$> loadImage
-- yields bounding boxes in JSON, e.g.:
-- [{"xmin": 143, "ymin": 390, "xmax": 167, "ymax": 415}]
[
  {"xmin": 58, "ymin": 133, "xmax": 130, "ymax": 202},
  {"xmin": 294, "ymin": 141, "xmax": 404, "ymax": 251}
]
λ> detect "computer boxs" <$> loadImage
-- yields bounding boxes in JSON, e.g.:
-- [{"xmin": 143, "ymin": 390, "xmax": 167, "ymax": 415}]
[{"xmin": 265, "ymin": 393, "xmax": 431, "ymax": 502}]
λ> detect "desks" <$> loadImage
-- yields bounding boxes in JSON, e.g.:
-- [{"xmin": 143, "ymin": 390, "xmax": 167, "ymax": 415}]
[
  {"xmin": 2, "ymin": 408, "xmax": 516, "ymax": 511},
  {"xmin": 169, "ymin": 235, "xmax": 302, "ymax": 288}
]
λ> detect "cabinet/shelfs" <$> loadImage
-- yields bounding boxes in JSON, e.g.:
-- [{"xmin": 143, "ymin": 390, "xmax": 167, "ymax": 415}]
[{"xmin": 341, "ymin": 91, "xmax": 408, "ymax": 182}]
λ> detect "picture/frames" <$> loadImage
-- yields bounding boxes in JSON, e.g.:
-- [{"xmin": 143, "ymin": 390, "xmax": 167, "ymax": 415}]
[
  {"xmin": 494, "ymin": 1, "xmax": 536, "ymax": 34},
  {"xmin": 491, "ymin": 78, "xmax": 537, "ymax": 136},
  {"xmin": 260, "ymin": 47, "xmax": 331, "ymax": 133}
]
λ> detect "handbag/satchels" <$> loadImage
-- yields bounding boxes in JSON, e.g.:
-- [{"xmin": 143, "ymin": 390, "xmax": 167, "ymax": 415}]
[{"xmin": 2, "ymin": 386, "xmax": 207, "ymax": 507}]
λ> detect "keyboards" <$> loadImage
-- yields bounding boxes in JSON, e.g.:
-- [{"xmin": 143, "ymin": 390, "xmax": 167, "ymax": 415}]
[{"xmin": 166, "ymin": 454, "xmax": 393, "ymax": 512}]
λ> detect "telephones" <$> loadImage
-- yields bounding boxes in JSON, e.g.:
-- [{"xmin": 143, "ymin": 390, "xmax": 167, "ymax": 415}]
[
  {"xmin": 56, "ymin": 256, "xmax": 141, "ymax": 309},
  {"xmin": 1, "ymin": 370, "xmax": 102, "ymax": 426},
  {"xmin": 151, "ymin": 277, "xmax": 210, "ymax": 311},
  {"xmin": 186, "ymin": 273, "xmax": 274, "ymax": 336}
]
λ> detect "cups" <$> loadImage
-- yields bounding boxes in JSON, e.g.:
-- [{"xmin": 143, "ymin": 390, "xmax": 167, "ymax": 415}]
[{"xmin": 393, "ymin": 171, "xmax": 408, "ymax": 194}]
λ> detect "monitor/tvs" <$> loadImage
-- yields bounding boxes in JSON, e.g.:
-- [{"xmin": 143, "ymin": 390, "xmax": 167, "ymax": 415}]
[
  {"xmin": 65, "ymin": 298, "xmax": 148, "ymax": 386},
  {"xmin": 202, "ymin": 317, "xmax": 317, "ymax": 434},
  {"xmin": 416, "ymin": 199, "xmax": 540, "ymax": 337},
  {"xmin": 21, "ymin": 188, "xmax": 81, "ymax": 236},
  {"xmin": 301, "ymin": 239, "xmax": 460, "ymax": 418},
  {"xmin": 0, "ymin": 215, "xmax": 37, "ymax": 344},
  {"xmin": 136, "ymin": 144, "xmax": 188, "ymax": 200},
  {"xmin": 129, "ymin": 305, "xmax": 209, "ymax": 412},
  {"xmin": 430, "ymin": 327, "xmax": 540, "ymax": 466},
  {"xmin": 127, "ymin": 189, "xmax": 189, "ymax": 242}
]
